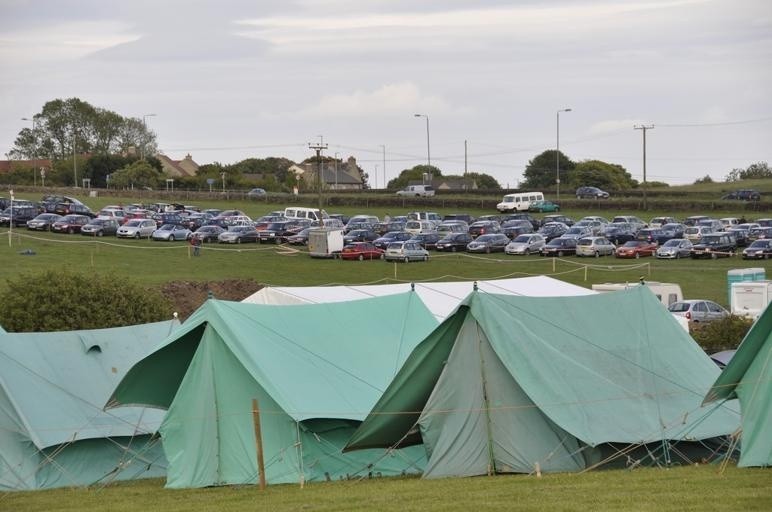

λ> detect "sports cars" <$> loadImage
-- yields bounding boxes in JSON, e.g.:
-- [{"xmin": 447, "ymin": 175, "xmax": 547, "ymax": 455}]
[{"xmin": 614, "ymin": 240, "xmax": 657, "ymax": 259}]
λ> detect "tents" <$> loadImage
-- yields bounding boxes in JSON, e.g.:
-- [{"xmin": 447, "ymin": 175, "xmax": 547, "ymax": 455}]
[
  {"xmin": 701, "ymin": 300, "xmax": 772, "ymax": 468},
  {"xmin": 339, "ymin": 282, "xmax": 741, "ymax": 480},
  {"xmin": 0, "ymin": 311, "xmax": 182, "ymax": 490},
  {"xmin": 102, "ymin": 281, "xmax": 438, "ymax": 490},
  {"xmin": 239, "ymin": 274, "xmax": 599, "ymax": 322}
]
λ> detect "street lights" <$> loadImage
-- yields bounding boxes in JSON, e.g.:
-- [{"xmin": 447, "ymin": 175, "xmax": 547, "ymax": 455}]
[
  {"xmin": 414, "ymin": 113, "xmax": 431, "ymax": 182},
  {"xmin": 555, "ymin": 108, "xmax": 573, "ymax": 200},
  {"xmin": 22, "ymin": 117, "xmax": 37, "ymax": 187},
  {"xmin": 142, "ymin": 112, "xmax": 160, "ymax": 125},
  {"xmin": 334, "ymin": 150, "xmax": 342, "ymax": 192}
]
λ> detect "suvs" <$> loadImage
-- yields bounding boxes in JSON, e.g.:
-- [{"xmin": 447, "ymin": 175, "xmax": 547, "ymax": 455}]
[
  {"xmin": 257, "ymin": 220, "xmax": 304, "ymax": 245},
  {"xmin": 690, "ymin": 232, "xmax": 738, "ymax": 260},
  {"xmin": 718, "ymin": 189, "xmax": 762, "ymax": 201}
]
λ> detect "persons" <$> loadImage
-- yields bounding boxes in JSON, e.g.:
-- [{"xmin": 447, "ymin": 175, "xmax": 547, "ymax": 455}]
[
  {"xmin": 193, "ymin": 233, "xmax": 200, "ymax": 256},
  {"xmin": 740, "ymin": 216, "xmax": 746, "ymax": 223},
  {"xmin": 384, "ymin": 213, "xmax": 391, "ymax": 223},
  {"xmin": 662, "ymin": 219, "xmax": 667, "ymax": 225}
]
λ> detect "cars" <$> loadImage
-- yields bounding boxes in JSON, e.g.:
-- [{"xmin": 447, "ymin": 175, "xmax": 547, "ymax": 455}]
[
  {"xmin": 434, "ymin": 233, "xmax": 474, "ymax": 253},
  {"xmin": 466, "ymin": 234, "xmax": 511, "ymax": 254},
  {"xmin": 52, "ymin": 214, "xmax": 91, "ymax": 234},
  {"xmin": 539, "ymin": 237, "xmax": 580, "ymax": 257},
  {"xmin": 152, "ymin": 223, "xmax": 193, "ymax": 242},
  {"xmin": 574, "ymin": 186, "xmax": 610, "ymax": 200},
  {"xmin": 742, "ymin": 238, "xmax": 772, "ymax": 260},
  {"xmin": 339, "ymin": 241, "xmax": 384, "ymax": 261},
  {"xmin": 218, "ymin": 226, "xmax": 260, "ymax": 245},
  {"xmin": 575, "ymin": 237, "xmax": 616, "ymax": 258},
  {"xmin": 286, "ymin": 227, "xmax": 319, "ymax": 246},
  {"xmin": 655, "ymin": 239, "xmax": 694, "ymax": 259},
  {"xmin": 383, "ymin": 240, "xmax": 430, "ymax": 263},
  {"xmin": 664, "ymin": 296, "xmax": 731, "ymax": 323},
  {"xmin": 116, "ymin": 218, "xmax": 158, "ymax": 240},
  {"xmin": 247, "ymin": 188, "xmax": 266, "ymax": 195},
  {"xmin": 190, "ymin": 225, "xmax": 227, "ymax": 244},
  {"xmin": 0, "ymin": 194, "xmax": 350, "ymax": 232},
  {"xmin": 342, "ymin": 210, "xmax": 772, "ymax": 249},
  {"xmin": 504, "ymin": 233, "xmax": 546, "ymax": 256},
  {"xmin": 80, "ymin": 218, "xmax": 121, "ymax": 237}
]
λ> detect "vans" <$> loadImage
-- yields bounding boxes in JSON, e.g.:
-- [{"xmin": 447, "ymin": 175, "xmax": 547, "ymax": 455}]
[
  {"xmin": 496, "ymin": 191, "xmax": 544, "ymax": 215},
  {"xmin": 395, "ymin": 185, "xmax": 435, "ymax": 198}
]
